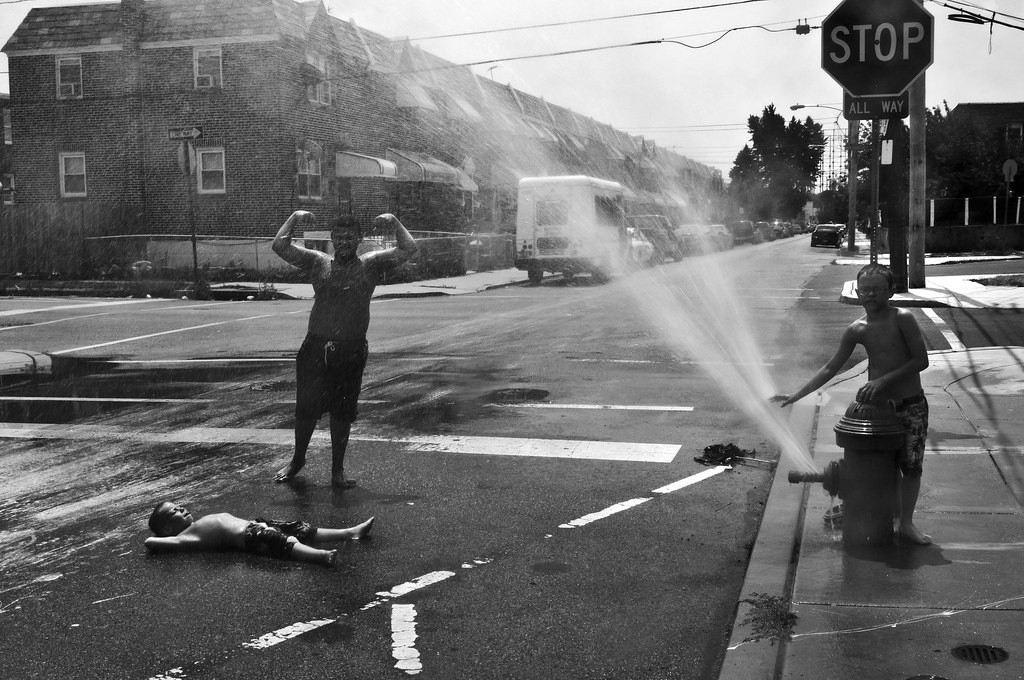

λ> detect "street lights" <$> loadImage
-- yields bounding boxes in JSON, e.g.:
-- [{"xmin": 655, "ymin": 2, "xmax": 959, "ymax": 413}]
[{"xmin": 788, "ymin": 103, "xmax": 859, "ymax": 255}]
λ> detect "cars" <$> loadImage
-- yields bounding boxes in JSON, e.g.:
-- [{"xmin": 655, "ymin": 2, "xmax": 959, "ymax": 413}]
[
  {"xmin": 810, "ymin": 224, "xmax": 847, "ymax": 249},
  {"xmin": 129, "ymin": 259, "xmax": 173, "ymax": 277},
  {"xmin": 624, "ymin": 213, "xmax": 802, "ymax": 265}
]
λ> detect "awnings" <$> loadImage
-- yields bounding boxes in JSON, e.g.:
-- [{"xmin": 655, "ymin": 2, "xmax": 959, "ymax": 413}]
[
  {"xmin": 447, "ymin": 98, "xmax": 481, "ymax": 122},
  {"xmin": 386, "ymin": 148, "xmax": 478, "ymax": 192},
  {"xmin": 335, "ymin": 151, "xmax": 398, "ymax": 178},
  {"xmin": 606, "ymin": 147, "xmax": 678, "ymax": 177},
  {"xmin": 397, "ymin": 81, "xmax": 436, "ymax": 110},
  {"xmin": 492, "ymin": 114, "xmax": 585, "ymax": 152},
  {"xmin": 622, "ymin": 187, "xmax": 687, "ymax": 208}
]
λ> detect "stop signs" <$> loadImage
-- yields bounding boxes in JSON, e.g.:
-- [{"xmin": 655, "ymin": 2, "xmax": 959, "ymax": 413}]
[{"xmin": 821, "ymin": 0, "xmax": 934, "ymax": 96}]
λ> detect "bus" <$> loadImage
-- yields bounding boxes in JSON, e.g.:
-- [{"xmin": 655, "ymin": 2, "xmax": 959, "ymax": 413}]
[{"xmin": 515, "ymin": 175, "xmax": 626, "ymax": 284}]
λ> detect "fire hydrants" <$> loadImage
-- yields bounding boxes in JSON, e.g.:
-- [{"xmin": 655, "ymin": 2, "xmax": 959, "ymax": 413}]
[{"xmin": 788, "ymin": 385, "xmax": 908, "ymax": 552}]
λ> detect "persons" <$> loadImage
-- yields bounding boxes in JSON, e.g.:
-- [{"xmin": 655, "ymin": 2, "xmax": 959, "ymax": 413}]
[
  {"xmin": 143, "ymin": 501, "xmax": 375, "ymax": 566},
  {"xmin": 272, "ymin": 209, "xmax": 417, "ymax": 489},
  {"xmin": 769, "ymin": 263, "xmax": 932, "ymax": 545}
]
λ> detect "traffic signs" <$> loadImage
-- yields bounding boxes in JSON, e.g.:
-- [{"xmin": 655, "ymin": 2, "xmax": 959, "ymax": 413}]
[{"xmin": 171, "ymin": 125, "xmax": 205, "ymax": 140}]
